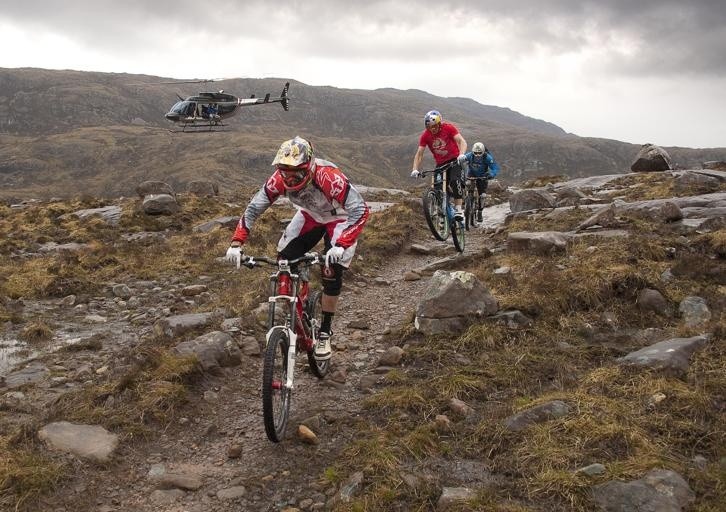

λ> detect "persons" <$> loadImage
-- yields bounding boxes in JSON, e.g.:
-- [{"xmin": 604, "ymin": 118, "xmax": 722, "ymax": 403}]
[
  {"xmin": 408, "ymin": 109, "xmax": 467, "ymax": 222},
  {"xmin": 226, "ymin": 136, "xmax": 369, "ymax": 360},
  {"xmin": 208, "ymin": 104, "xmax": 220, "ymax": 120},
  {"xmin": 463, "ymin": 140, "xmax": 499, "ymax": 222}
]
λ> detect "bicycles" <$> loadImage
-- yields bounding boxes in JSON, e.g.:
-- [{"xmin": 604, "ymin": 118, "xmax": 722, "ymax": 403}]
[
  {"xmin": 418, "ymin": 157, "xmax": 494, "ymax": 252},
  {"xmin": 240, "ymin": 252, "xmax": 343, "ymax": 444}
]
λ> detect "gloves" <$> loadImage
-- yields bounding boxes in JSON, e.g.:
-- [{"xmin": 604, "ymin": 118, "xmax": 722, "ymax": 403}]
[
  {"xmin": 226, "ymin": 245, "xmax": 244, "ymax": 269},
  {"xmin": 410, "ymin": 171, "xmax": 419, "ymax": 180},
  {"xmin": 457, "ymin": 155, "xmax": 465, "ymax": 165},
  {"xmin": 485, "ymin": 172, "xmax": 492, "ymax": 180},
  {"xmin": 325, "ymin": 246, "xmax": 345, "ymax": 268}
]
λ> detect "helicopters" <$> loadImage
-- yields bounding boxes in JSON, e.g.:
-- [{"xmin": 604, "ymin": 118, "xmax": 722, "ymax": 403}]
[{"xmin": 126, "ymin": 77, "xmax": 291, "ymax": 134}]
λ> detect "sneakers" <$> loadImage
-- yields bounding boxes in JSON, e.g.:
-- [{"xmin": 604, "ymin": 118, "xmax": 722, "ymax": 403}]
[
  {"xmin": 453, "ymin": 211, "xmax": 464, "ymax": 221},
  {"xmin": 477, "ymin": 211, "xmax": 483, "ymax": 221},
  {"xmin": 314, "ymin": 330, "xmax": 332, "ymax": 361},
  {"xmin": 439, "ymin": 223, "xmax": 445, "ymax": 233}
]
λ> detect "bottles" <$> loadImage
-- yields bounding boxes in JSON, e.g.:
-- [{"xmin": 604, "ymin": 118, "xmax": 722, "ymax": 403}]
[{"xmin": 436, "ymin": 173, "xmax": 441, "ymax": 190}]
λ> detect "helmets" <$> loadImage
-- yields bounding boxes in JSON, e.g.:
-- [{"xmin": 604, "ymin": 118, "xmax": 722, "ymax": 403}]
[
  {"xmin": 425, "ymin": 110, "xmax": 443, "ymax": 128},
  {"xmin": 272, "ymin": 137, "xmax": 314, "ymax": 192},
  {"xmin": 472, "ymin": 142, "xmax": 485, "ymax": 157}
]
